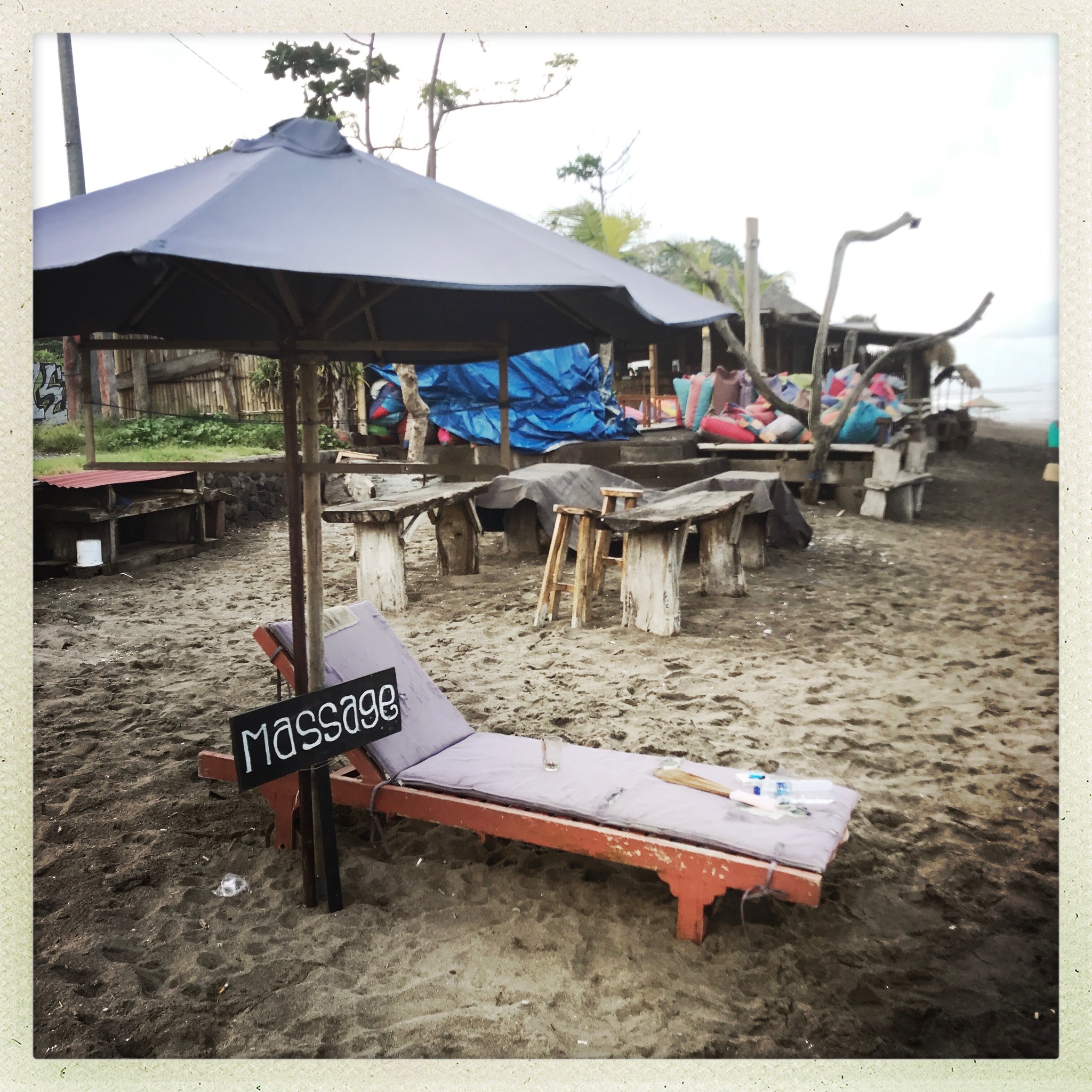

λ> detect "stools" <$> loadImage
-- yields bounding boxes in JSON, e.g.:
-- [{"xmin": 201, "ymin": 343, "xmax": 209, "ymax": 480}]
[
  {"xmin": 617, "ymin": 394, "xmax": 681, "ymax": 425},
  {"xmin": 532, "ymin": 504, "xmax": 601, "ymax": 630},
  {"xmin": 591, "ymin": 486, "xmax": 644, "ymax": 596}
]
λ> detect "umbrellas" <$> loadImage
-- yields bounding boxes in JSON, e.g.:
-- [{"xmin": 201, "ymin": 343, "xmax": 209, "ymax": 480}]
[{"xmin": 32, "ymin": 118, "xmax": 745, "ymax": 895}]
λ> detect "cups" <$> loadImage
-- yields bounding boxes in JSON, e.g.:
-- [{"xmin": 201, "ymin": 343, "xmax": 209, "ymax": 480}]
[{"xmin": 541, "ymin": 735, "xmax": 563, "ymax": 771}]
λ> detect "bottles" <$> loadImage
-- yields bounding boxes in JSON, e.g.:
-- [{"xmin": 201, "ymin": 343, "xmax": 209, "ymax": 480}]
[{"xmin": 754, "ymin": 779, "xmax": 834, "ymax": 804}]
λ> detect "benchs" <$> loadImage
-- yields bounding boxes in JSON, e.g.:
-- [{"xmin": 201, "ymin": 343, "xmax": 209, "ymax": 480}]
[
  {"xmin": 714, "ymin": 470, "xmax": 779, "ymax": 570},
  {"xmin": 494, "ymin": 462, "xmax": 594, "ymax": 556},
  {"xmin": 594, "ymin": 490, "xmax": 752, "ymax": 635},
  {"xmin": 859, "ymin": 440, "xmax": 933, "ymax": 523},
  {"xmin": 319, "ymin": 481, "xmax": 490, "ymax": 614},
  {"xmin": 198, "ymin": 600, "xmax": 860, "ymax": 946}
]
